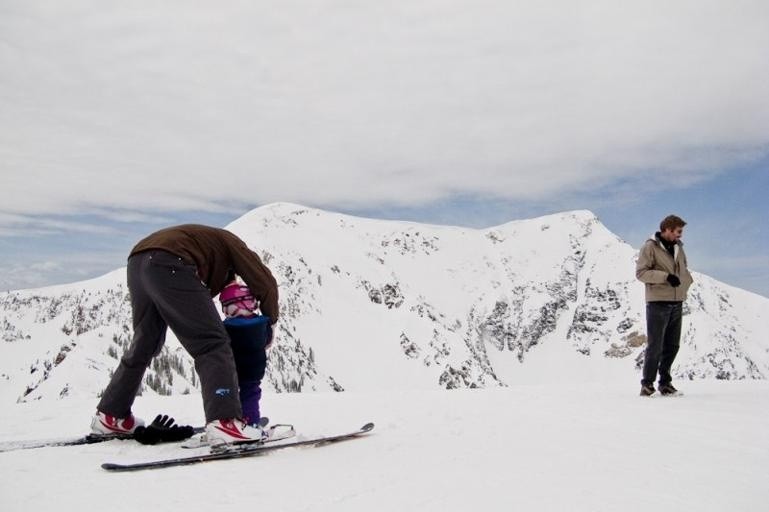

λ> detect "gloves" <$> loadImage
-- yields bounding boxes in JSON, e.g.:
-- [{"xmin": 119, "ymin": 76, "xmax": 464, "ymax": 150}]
[
  {"xmin": 666, "ymin": 273, "xmax": 681, "ymax": 287},
  {"xmin": 133, "ymin": 414, "xmax": 195, "ymax": 445}
]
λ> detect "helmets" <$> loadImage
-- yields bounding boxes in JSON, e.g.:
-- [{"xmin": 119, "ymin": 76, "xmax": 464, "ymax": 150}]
[{"xmin": 218, "ymin": 284, "xmax": 259, "ymax": 318}]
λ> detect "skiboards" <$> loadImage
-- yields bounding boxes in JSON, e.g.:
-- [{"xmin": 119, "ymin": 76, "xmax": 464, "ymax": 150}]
[
  {"xmin": 0, "ymin": 422, "xmax": 373, "ymax": 472},
  {"xmin": 181, "ymin": 423, "xmax": 296, "ymax": 449}
]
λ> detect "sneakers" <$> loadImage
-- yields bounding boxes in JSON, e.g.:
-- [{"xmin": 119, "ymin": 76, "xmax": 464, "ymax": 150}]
[
  {"xmin": 658, "ymin": 382, "xmax": 683, "ymax": 395},
  {"xmin": 90, "ymin": 408, "xmax": 147, "ymax": 434},
  {"xmin": 641, "ymin": 382, "xmax": 654, "ymax": 395},
  {"xmin": 205, "ymin": 417, "xmax": 265, "ymax": 447}
]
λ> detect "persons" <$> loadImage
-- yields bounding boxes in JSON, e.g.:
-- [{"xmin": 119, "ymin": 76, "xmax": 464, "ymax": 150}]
[
  {"xmin": 219, "ymin": 283, "xmax": 273, "ymax": 427},
  {"xmin": 636, "ymin": 215, "xmax": 693, "ymax": 397},
  {"xmin": 91, "ymin": 225, "xmax": 279, "ymax": 448}
]
